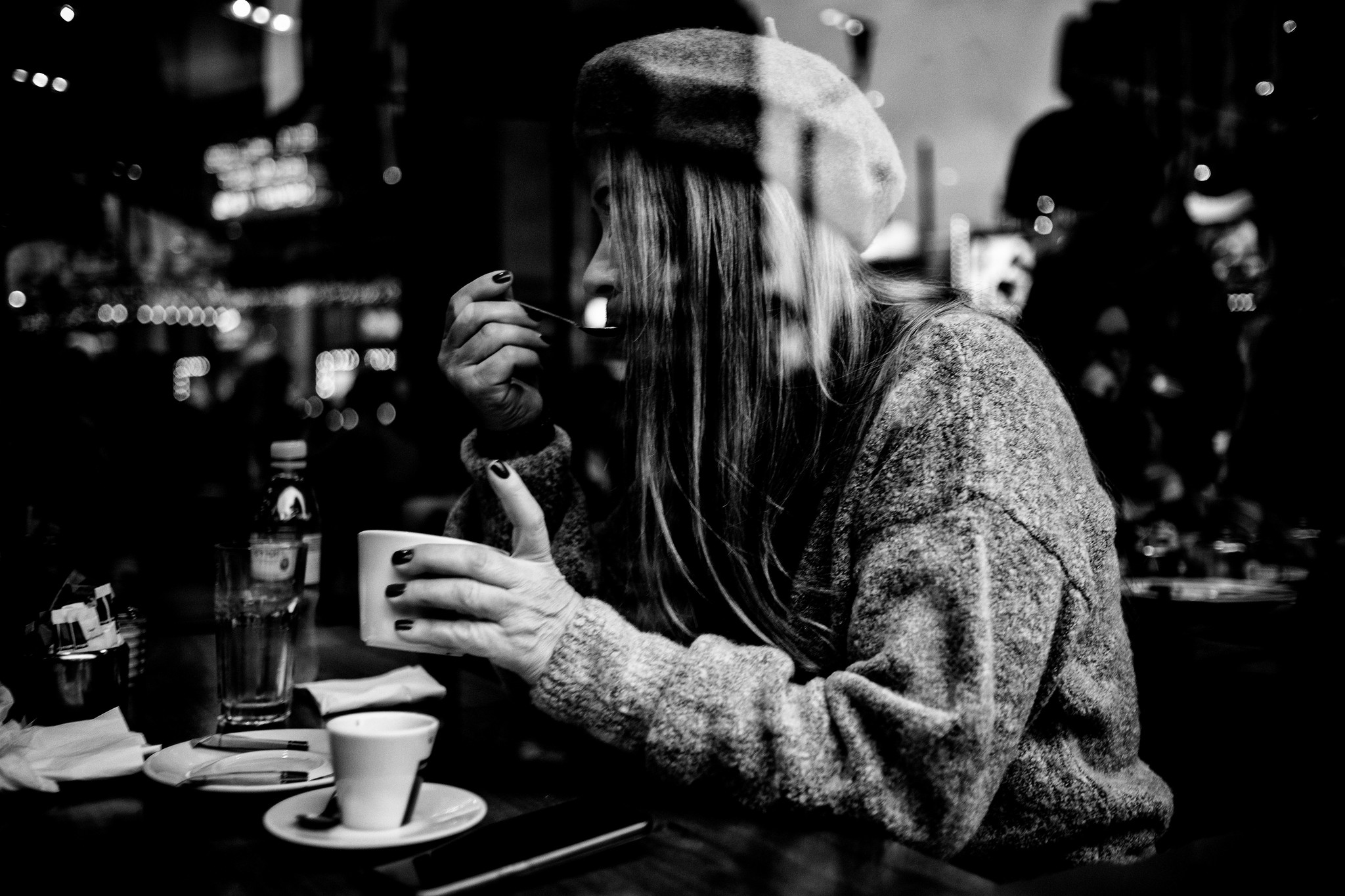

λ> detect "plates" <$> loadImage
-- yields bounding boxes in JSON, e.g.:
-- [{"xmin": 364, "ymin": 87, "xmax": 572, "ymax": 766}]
[
  {"xmin": 263, "ymin": 782, "xmax": 488, "ymax": 849},
  {"xmin": 143, "ymin": 728, "xmax": 340, "ymax": 792}
]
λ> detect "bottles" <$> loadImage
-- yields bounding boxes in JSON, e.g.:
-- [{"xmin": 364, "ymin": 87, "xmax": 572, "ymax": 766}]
[{"xmin": 252, "ymin": 439, "xmax": 320, "ymax": 684}]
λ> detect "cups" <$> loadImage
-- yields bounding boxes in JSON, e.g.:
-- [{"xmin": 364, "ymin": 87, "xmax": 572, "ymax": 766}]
[
  {"xmin": 359, "ymin": 530, "xmax": 516, "ymax": 658},
  {"xmin": 326, "ymin": 711, "xmax": 440, "ymax": 830},
  {"xmin": 213, "ymin": 537, "xmax": 310, "ymax": 729}
]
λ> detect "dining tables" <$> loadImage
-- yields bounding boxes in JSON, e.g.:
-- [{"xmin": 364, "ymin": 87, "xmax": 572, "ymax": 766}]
[{"xmin": 0, "ymin": 578, "xmax": 1008, "ymax": 896}]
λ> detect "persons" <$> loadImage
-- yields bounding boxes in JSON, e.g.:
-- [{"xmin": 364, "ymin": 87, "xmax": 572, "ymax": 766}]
[{"xmin": 382, "ymin": 28, "xmax": 1179, "ymax": 895}]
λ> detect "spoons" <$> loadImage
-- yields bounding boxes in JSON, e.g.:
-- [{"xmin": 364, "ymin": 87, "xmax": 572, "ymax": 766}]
[
  {"xmin": 500, "ymin": 295, "xmax": 620, "ymax": 338},
  {"xmin": 296, "ymin": 787, "xmax": 340, "ymax": 830}
]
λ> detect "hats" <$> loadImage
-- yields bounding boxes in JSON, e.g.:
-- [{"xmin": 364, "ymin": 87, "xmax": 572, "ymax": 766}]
[{"xmin": 577, "ymin": 27, "xmax": 907, "ymax": 253}]
